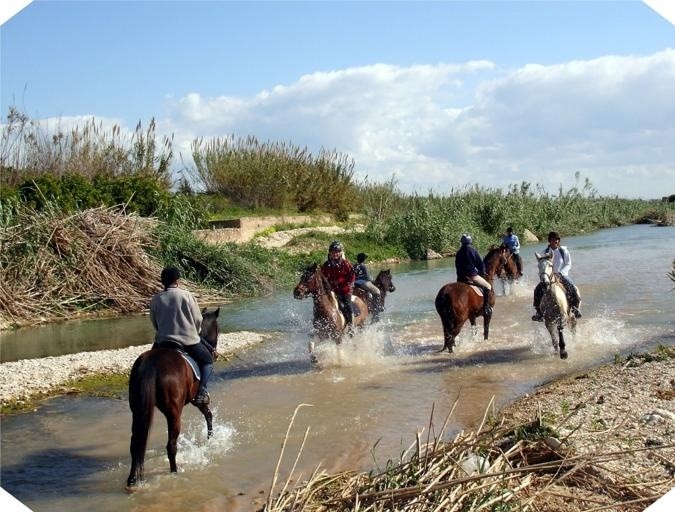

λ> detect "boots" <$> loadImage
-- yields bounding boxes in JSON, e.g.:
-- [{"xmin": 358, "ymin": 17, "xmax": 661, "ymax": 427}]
[{"xmin": 484, "ymin": 289, "xmax": 492, "ymax": 312}]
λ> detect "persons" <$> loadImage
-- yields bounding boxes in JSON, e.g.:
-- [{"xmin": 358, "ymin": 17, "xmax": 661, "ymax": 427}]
[
  {"xmin": 455, "ymin": 234, "xmax": 493, "ymax": 316},
  {"xmin": 503, "ymin": 227, "xmax": 524, "ymax": 276},
  {"xmin": 353, "ymin": 253, "xmax": 382, "ymax": 312},
  {"xmin": 149, "ymin": 266, "xmax": 214, "ymax": 404},
  {"xmin": 531, "ymin": 231, "xmax": 582, "ymax": 321},
  {"xmin": 322, "ymin": 240, "xmax": 355, "ymax": 337}
]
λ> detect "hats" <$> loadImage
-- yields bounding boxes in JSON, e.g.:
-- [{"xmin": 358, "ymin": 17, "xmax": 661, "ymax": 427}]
[
  {"xmin": 462, "ymin": 235, "xmax": 472, "ymax": 243},
  {"xmin": 161, "ymin": 268, "xmax": 180, "ymax": 285},
  {"xmin": 330, "ymin": 241, "xmax": 342, "ymax": 252},
  {"xmin": 358, "ymin": 253, "xmax": 368, "ymax": 263},
  {"xmin": 549, "ymin": 232, "xmax": 561, "ymax": 239}
]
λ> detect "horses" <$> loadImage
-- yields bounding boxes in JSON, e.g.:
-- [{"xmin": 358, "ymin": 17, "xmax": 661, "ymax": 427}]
[
  {"xmin": 354, "ymin": 267, "xmax": 397, "ymax": 324},
  {"xmin": 127, "ymin": 306, "xmax": 221, "ymax": 485},
  {"xmin": 293, "ymin": 261, "xmax": 370, "ymax": 370},
  {"xmin": 498, "ymin": 239, "xmax": 523, "ymax": 298},
  {"xmin": 529, "ymin": 249, "xmax": 582, "ymax": 359},
  {"xmin": 434, "ymin": 243, "xmax": 508, "ymax": 354}
]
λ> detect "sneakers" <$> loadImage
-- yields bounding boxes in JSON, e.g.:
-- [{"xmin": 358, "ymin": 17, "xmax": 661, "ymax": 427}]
[
  {"xmin": 532, "ymin": 316, "xmax": 543, "ymax": 321},
  {"xmin": 195, "ymin": 395, "xmax": 209, "ymax": 404},
  {"xmin": 575, "ymin": 310, "xmax": 581, "ymax": 318}
]
